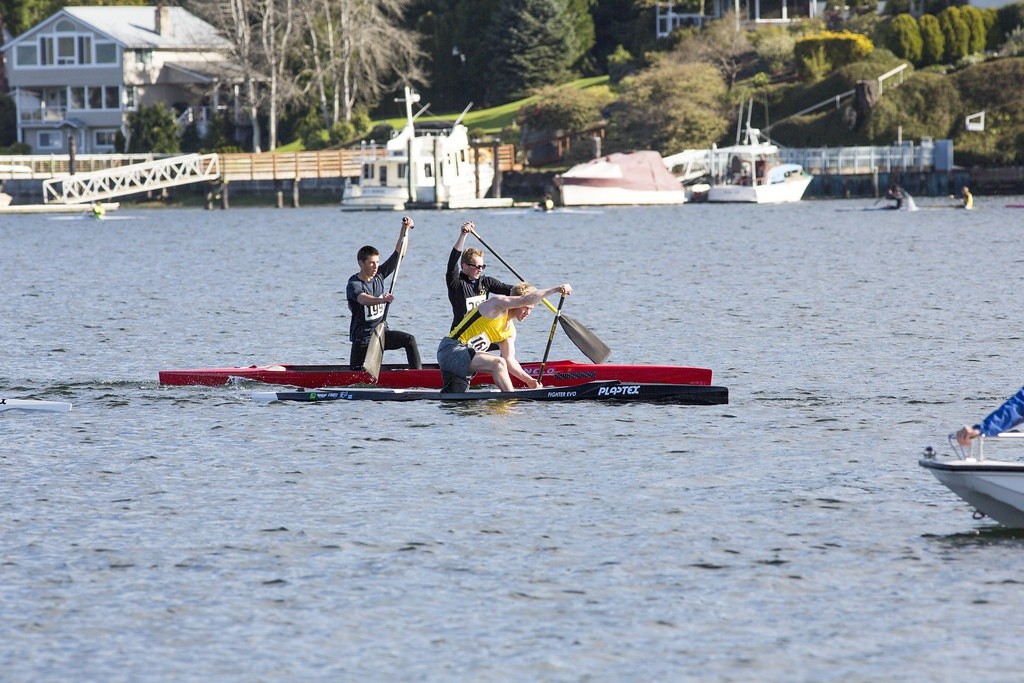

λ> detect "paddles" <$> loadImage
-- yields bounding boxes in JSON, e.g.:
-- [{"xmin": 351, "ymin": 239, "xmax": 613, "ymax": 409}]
[
  {"xmin": 362, "ymin": 216, "xmax": 415, "ymax": 383},
  {"xmin": 537, "ymin": 289, "xmax": 568, "ymax": 386},
  {"xmin": 463, "ymin": 220, "xmax": 612, "ymax": 365}
]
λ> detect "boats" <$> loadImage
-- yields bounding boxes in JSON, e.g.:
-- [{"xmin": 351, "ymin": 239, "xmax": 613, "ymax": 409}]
[
  {"xmin": 159, "ymin": 358, "xmax": 711, "ymax": 390},
  {"xmin": 341, "ymin": 102, "xmax": 494, "ymax": 213},
  {"xmin": 553, "ymin": 92, "xmax": 814, "ymax": 209},
  {"xmin": 919, "ymin": 433, "xmax": 1024, "ymax": 529},
  {"xmin": 253, "ymin": 379, "xmax": 728, "ymax": 406}
]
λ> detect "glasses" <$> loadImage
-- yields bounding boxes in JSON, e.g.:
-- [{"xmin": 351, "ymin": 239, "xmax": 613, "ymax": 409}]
[{"xmin": 466, "ymin": 263, "xmax": 486, "ymax": 270}]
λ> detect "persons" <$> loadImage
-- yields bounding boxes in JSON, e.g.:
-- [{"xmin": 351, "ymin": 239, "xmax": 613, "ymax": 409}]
[
  {"xmin": 955, "ymin": 385, "xmax": 1024, "ymax": 445},
  {"xmin": 445, "ymin": 221, "xmax": 514, "ymax": 352},
  {"xmin": 94, "ymin": 202, "xmax": 105, "ymax": 217},
  {"xmin": 886, "ymin": 183, "xmax": 909, "ymax": 206},
  {"xmin": 950, "ymin": 186, "xmax": 973, "ymax": 209},
  {"xmin": 436, "ymin": 282, "xmax": 572, "ymax": 391},
  {"xmin": 538, "ymin": 194, "xmax": 554, "ymax": 210},
  {"xmin": 346, "ymin": 217, "xmax": 422, "ymax": 371}
]
